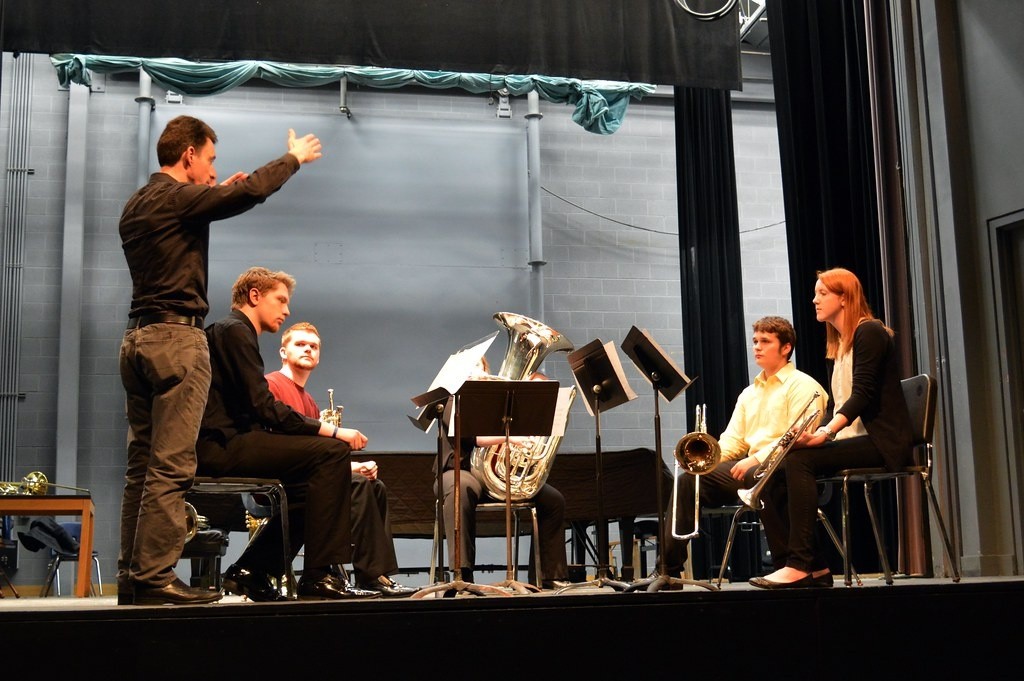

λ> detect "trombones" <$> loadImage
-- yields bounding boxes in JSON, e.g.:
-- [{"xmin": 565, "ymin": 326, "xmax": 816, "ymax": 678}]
[{"xmin": 671, "ymin": 404, "xmax": 727, "ymax": 540}]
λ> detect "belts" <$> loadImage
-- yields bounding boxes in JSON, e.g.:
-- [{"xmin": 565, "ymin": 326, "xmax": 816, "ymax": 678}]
[{"xmin": 127, "ymin": 311, "xmax": 205, "ymax": 329}]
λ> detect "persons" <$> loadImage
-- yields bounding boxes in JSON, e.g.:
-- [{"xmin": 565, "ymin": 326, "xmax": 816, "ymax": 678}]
[
  {"xmin": 748, "ymin": 270, "xmax": 912, "ymax": 589},
  {"xmin": 263, "ymin": 322, "xmax": 421, "ymax": 597},
  {"xmin": 198, "ymin": 269, "xmax": 380, "ymax": 604},
  {"xmin": 118, "ymin": 116, "xmax": 323, "ymax": 604},
  {"xmin": 632, "ymin": 315, "xmax": 828, "ymax": 591},
  {"xmin": 431, "ymin": 359, "xmax": 568, "ymax": 590}
]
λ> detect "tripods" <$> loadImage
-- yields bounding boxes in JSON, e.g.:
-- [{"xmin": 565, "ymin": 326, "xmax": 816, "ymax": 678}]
[{"xmin": 407, "ymin": 324, "xmax": 722, "ymax": 599}]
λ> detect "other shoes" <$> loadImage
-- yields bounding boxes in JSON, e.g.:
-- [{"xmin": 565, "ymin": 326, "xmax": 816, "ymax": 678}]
[
  {"xmin": 747, "ymin": 568, "xmax": 835, "ymax": 587},
  {"xmin": 634, "ymin": 571, "xmax": 685, "ymax": 591}
]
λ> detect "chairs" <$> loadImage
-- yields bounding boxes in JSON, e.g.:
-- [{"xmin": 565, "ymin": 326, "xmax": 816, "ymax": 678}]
[
  {"xmin": 814, "ymin": 374, "xmax": 962, "ymax": 587},
  {"xmin": 186, "ymin": 475, "xmax": 302, "ymax": 597},
  {"xmin": 52, "ymin": 522, "xmax": 103, "ymax": 596},
  {"xmin": 429, "ymin": 495, "xmax": 544, "ymax": 594},
  {"xmin": 701, "ymin": 480, "xmax": 863, "ymax": 589}
]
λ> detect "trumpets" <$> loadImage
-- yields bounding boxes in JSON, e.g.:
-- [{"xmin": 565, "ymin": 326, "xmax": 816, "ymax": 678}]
[
  {"xmin": 0, "ymin": 470, "xmax": 91, "ymax": 495},
  {"xmin": 181, "ymin": 498, "xmax": 210, "ymax": 543},
  {"xmin": 320, "ymin": 387, "xmax": 343, "ymax": 428},
  {"xmin": 737, "ymin": 391, "xmax": 819, "ymax": 510}
]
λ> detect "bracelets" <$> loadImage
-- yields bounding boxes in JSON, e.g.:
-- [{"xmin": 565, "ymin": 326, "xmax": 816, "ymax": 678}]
[
  {"xmin": 820, "ymin": 425, "xmax": 837, "ymax": 441},
  {"xmin": 331, "ymin": 426, "xmax": 338, "ymax": 439}
]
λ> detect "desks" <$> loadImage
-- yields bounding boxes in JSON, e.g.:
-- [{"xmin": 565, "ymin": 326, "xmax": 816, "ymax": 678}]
[{"xmin": 0, "ymin": 494, "xmax": 95, "ymax": 597}]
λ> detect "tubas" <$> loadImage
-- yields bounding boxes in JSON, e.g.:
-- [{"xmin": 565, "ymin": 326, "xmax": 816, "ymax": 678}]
[{"xmin": 470, "ymin": 311, "xmax": 578, "ymax": 503}]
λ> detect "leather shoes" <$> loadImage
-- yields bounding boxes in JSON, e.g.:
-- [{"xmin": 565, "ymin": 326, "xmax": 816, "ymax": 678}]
[
  {"xmin": 225, "ymin": 564, "xmax": 295, "ymax": 602},
  {"xmin": 355, "ymin": 575, "xmax": 424, "ymax": 598},
  {"xmin": 297, "ymin": 570, "xmax": 382, "ymax": 598},
  {"xmin": 117, "ymin": 576, "xmax": 223, "ymax": 608}
]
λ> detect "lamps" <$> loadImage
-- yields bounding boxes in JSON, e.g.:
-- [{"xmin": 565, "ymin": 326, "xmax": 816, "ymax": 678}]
[
  {"xmin": 496, "ymin": 96, "xmax": 512, "ymax": 119},
  {"xmin": 165, "ymin": 88, "xmax": 184, "ymax": 105}
]
